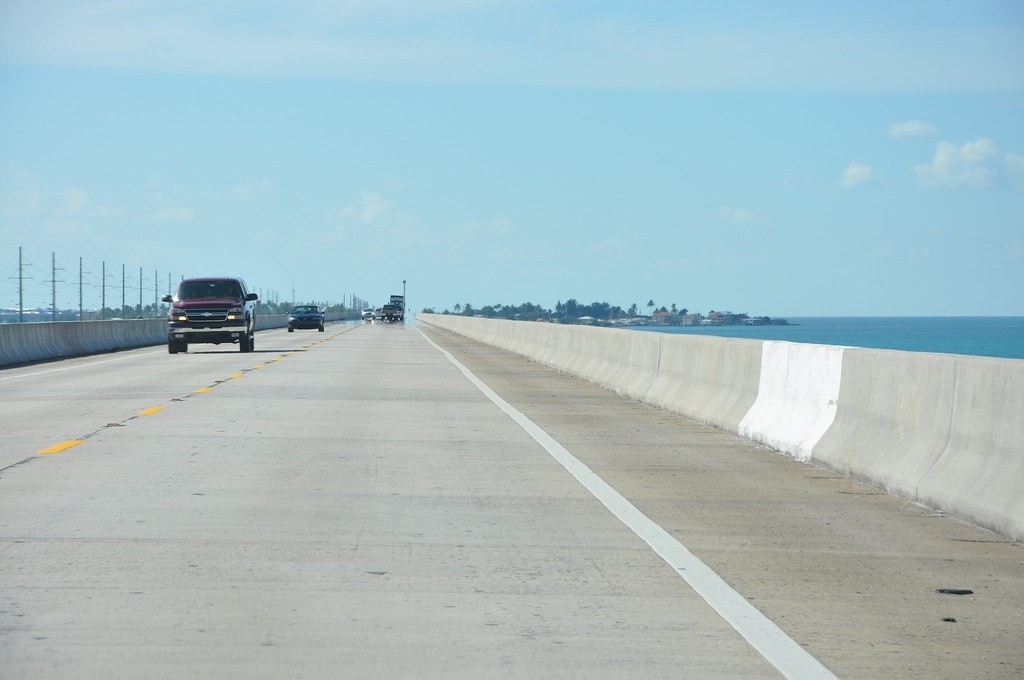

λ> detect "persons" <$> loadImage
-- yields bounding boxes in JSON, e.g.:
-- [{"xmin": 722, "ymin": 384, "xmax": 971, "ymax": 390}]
[
  {"xmin": 227, "ymin": 283, "xmax": 241, "ymax": 297},
  {"xmin": 190, "ymin": 288, "xmax": 202, "ymax": 297}
]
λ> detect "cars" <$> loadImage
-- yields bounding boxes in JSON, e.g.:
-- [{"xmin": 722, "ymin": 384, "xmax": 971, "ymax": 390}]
[
  {"xmin": 361, "ymin": 308, "xmax": 377, "ymax": 320},
  {"xmin": 161, "ymin": 278, "xmax": 258, "ymax": 353},
  {"xmin": 286, "ymin": 306, "xmax": 326, "ymax": 332},
  {"xmin": 375, "ymin": 308, "xmax": 382, "ymax": 317}
]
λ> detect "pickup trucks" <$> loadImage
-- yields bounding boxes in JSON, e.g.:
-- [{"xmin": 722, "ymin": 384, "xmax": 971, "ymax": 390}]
[
  {"xmin": 381, "ymin": 305, "xmax": 398, "ymax": 321},
  {"xmin": 396, "ymin": 305, "xmax": 405, "ymax": 320}
]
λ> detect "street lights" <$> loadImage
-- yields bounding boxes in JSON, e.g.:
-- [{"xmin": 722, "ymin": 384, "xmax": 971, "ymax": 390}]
[{"xmin": 402, "ymin": 280, "xmax": 406, "ymax": 296}]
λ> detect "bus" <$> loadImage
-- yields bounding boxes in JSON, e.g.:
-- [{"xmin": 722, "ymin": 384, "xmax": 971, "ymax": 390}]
[{"xmin": 390, "ymin": 295, "xmax": 404, "ymax": 309}]
